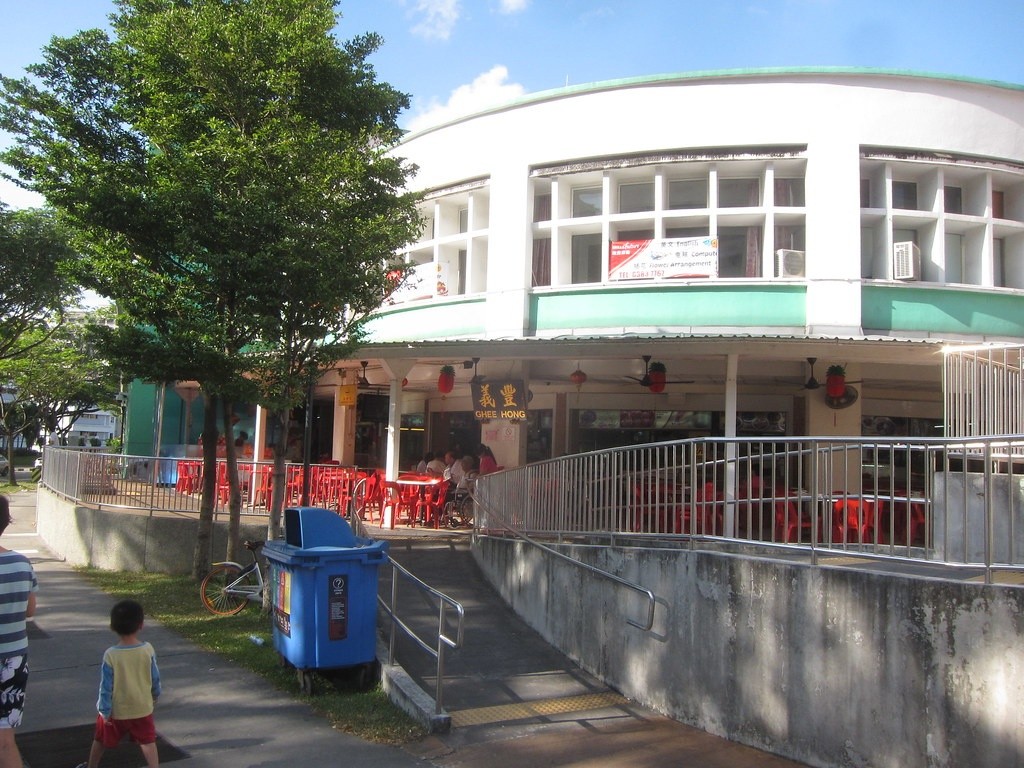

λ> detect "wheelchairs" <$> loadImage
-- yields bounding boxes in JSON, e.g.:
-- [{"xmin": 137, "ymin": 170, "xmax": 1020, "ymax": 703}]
[{"xmin": 446, "ymin": 477, "xmax": 488, "ymax": 528}]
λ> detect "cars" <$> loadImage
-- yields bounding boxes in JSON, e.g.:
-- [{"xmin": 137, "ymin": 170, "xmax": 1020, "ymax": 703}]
[
  {"xmin": 35, "ymin": 456, "xmax": 43, "ymax": 469},
  {"xmin": 0, "ymin": 454, "xmax": 9, "ymax": 476}
]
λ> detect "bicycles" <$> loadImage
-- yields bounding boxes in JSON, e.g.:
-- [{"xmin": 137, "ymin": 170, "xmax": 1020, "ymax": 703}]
[{"xmin": 198, "ymin": 538, "xmax": 267, "ymax": 616}]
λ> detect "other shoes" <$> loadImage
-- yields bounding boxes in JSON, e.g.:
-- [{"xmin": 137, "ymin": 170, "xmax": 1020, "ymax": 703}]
[{"xmin": 72, "ymin": 759, "xmax": 88, "ymax": 768}]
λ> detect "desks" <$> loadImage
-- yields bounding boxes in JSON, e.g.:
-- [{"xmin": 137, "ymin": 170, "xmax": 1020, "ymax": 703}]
[{"xmin": 395, "ymin": 479, "xmax": 445, "ymax": 528}]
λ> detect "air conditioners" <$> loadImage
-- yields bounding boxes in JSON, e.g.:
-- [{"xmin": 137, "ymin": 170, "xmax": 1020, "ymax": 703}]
[
  {"xmin": 773, "ymin": 249, "xmax": 805, "ymax": 277},
  {"xmin": 893, "ymin": 241, "xmax": 921, "ymax": 281}
]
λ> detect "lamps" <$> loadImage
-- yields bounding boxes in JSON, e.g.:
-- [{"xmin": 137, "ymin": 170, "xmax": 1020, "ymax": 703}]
[
  {"xmin": 826, "ymin": 366, "xmax": 845, "ymax": 398},
  {"xmin": 438, "ymin": 366, "xmax": 455, "ymax": 395},
  {"xmin": 571, "ymin": 361, "xmax": 587, "ymax": 393},
  {"xmin": 648, "ymin": 361, "xmax": 666, "ymax": 393}
]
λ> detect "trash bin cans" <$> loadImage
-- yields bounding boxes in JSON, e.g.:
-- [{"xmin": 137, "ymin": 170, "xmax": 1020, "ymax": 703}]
[{"xmin": 262, "ymin": 506, "xmax": 390, "ymax": 699}]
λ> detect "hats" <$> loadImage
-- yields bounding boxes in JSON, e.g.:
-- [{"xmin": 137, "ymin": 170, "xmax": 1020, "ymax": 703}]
[
  {"xmin": 240, "ymin": 432, "xmax": 248, "ymax": 439},
  {"xmin": 459, "ymin": 456, "xmax": 474, "ymax": 465}
]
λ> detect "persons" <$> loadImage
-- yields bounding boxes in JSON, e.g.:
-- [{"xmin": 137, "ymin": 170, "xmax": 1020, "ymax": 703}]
[
  {"xmin": 236, "ymin": 431, "xmax": 248, "ymax": 448},
  {"xmin": 0, "ymin": 494, "xmax": 38, "ymax": 768},
  {"xmin": 415, "ymin": 442, "xmax": 497, "ymax": 527},
  {"xmin": 87, "ymin": 599, "xmax": 162, "ymax": 768},
  {"xmin": 48, "ymin": 425, "xmax": 59, "ymax": 446}
]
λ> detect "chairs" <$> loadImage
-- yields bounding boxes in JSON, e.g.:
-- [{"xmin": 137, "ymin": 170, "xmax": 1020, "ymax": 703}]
[
  {"xmin": 634, "ymin": 471, "xmax": 932, "ymax": 552},
  {"xmin": 175, "ymin": 457, "xmax": 453, "ymax": 530}
]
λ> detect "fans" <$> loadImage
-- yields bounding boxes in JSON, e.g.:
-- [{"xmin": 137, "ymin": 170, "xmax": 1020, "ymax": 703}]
[
  {"xmin": 621, "ymin": 355, "xmax": 696, "ymax": 388},
  {"xmin": 355, "ymin": 361, "xmax": 385, "ymax": 388},
  {"xmin": 773, "ymin": 358, "xmax": 864, "ymax": 391}
]
